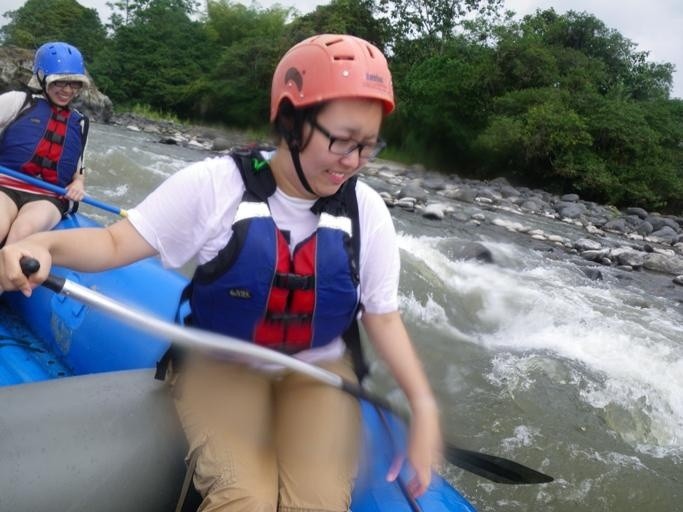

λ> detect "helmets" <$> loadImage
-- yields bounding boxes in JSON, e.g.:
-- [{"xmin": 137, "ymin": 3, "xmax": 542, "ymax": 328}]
[
  {"xmin": 33, "ymin": 42, "xmax": 85, "ymax": 93},
  {"xmin": 271, "ymin": 34, "xmax": 395, "ymax": 122}
]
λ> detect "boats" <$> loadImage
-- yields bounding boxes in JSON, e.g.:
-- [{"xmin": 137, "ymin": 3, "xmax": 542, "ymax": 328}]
[{"xmin": 0, "ymin": 211, "xmax": 476, "ymax": 512}]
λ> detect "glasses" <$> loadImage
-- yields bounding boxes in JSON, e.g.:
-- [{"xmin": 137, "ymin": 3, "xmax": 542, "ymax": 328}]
[
  {"xmin": 303, "ymin": 114, "xmax": 388, "ymax": 157},
  {"xmin": 53, "ymin": 81, "xmax": 82, "ymax": 89}
]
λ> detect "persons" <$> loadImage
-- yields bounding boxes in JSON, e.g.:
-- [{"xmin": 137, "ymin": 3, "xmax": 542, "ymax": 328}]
[
  {"xmin": 0, "ymin": 33, "xmax": 444, "ymax": 511},
  {"xmin": 1, "ymin": 40, "xmax": 89, "ymax": 300}
]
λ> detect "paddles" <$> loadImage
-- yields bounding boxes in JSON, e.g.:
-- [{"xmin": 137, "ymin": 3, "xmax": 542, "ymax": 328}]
[{"xmin": 19, "ymin": 258, "xmax": 553, "ymax": 484}]
